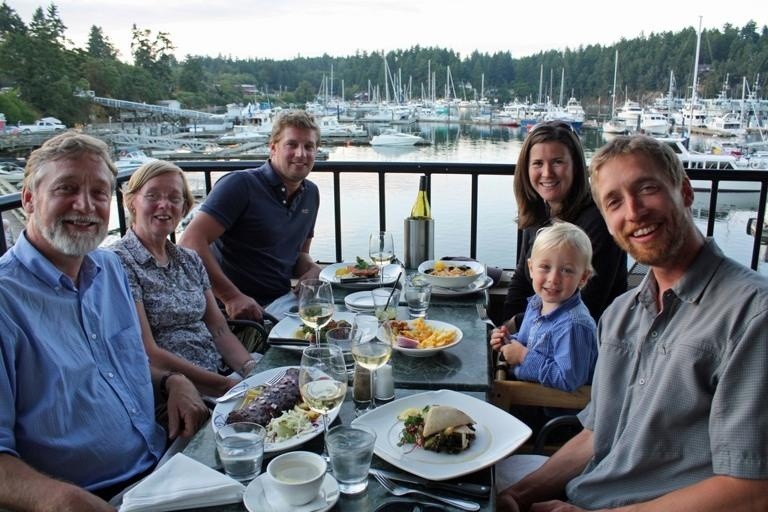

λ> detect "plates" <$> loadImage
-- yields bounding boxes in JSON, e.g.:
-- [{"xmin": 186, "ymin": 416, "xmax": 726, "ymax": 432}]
[
  {"xmin": 344, "ymin": 291, "xmax": 393, "ymax": 313},
  {"xmin": 350, "ymin": 389, "xmax": 533, "ymax": 482},
  {"xmin": 409, "ymin": 273, "xmax": 494, "ymax": 296},
  {"xmin": 211, "ymin": 366, "xmax": 344, "ymax": 453},
  {"xmin": 242, "ymin": 473, "xmax": 340, "ymax": 512},
  {"xmin": 318, "ymin": 262, "xmax": 402, "ymax": 289},
  {"xmin": 267, "ymin": 311, "xmax": 379, "ymax": 354}
]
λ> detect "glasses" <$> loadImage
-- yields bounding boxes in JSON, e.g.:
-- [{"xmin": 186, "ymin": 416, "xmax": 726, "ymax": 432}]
[
  {"xmin": 136, "ymin": 189, "xmax": 182, "ymax": 203},
  {"xmin": 529, "ymin": 121, "xmax": 575, "ymax": 134}
]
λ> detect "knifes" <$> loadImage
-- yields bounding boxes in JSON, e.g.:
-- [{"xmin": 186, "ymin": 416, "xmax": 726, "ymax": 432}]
[{"xmin": 367, "ymin": 467, "xmax": 492, "ymax": 495}]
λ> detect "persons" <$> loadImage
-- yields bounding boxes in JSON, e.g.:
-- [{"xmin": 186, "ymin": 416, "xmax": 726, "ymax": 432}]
[
  {"xmin": 491, "ymin": 221, "xmax": 598, "ymax": 391},
  {"xmin": 500, "ymin": 137, "xmax": 768, "ymax": 512},
  {"xmin": 498, "ymin": 119, "xmax": 628, "ymax": 323},
  {"xmin": 1, "ymin": 130, "xmax": 209, "ymax": 511},
  {"xmin": 108, "ymin": 160, "xmax": 258, "ymax": 396},
  {"xmin": 178, "ymin": 109, "xmax": 322, "ymax": 353}
]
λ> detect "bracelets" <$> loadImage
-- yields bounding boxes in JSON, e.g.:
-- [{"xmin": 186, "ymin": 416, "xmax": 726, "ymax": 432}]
[{"xmin": 240, "ymin": 358, "xmax": 255, "ymax": 375}]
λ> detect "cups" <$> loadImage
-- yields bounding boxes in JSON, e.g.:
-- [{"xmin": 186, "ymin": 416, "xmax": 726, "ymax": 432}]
[
  {"xmin": 298, "ymin": 279, "xmax": 334, "ymax": 374},
  {"xmin": 404, "ymin": 282, "xmax": 432, "ymax": 318},
  {"xmin": 372, "ymin": 287, "xmax": 400, "ymax": 323},
  {"xmin": 326, "ymin": 327, "xmax": 359, "ymax": 373},
  {"xmin": 326, "ymin": 424, "xmax": 378, "ymax": 496},
  {"xmin": 298, "ymin": 344, "xmax": 348, "ymax": 473},
  {"xmin": 369, "ymin": 231, "xmax": 394, "ymax": 289},
  {"xmin": 350, "ymin": 310, "xmax": 393, "ymax": 420},
  {"xmin": 381, "ymin": 263, "xmax": 406, "ymax": 319},
  {"xmin": 215, "ymin": 422, "xmax": 266, "ymax": 481}
]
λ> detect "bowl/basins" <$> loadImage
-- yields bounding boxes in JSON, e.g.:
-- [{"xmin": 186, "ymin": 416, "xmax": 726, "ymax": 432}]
[
  {"xmin": 376, "ymin": 319, "xmax": 464, "ymax": 358},
  {"xmin": 418, "ymin": 260, "xmax": 485, "ymax": 288},
  {"xmin": 267, "ymin": 450, "xmax": 327, "ymax": 506}
]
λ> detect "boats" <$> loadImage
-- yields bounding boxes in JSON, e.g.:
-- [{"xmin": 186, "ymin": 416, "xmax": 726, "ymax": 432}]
[
  {"xmin": 368, "ymin": 128, "xmax": 423, "ymax": 146},
  {"xmin": 0, "ymin": 160, "xmax": 26, "ymax": 184},
  {"xmin": 654, "ymin": 137, "xmax": 761, "ymax": 192},
  {"xmin": 503, "ymin": 98, "xmax": 527, "ymax": 122},
  {"xmin": 320, "ymin": 116, "xmax": 368, "ymax": 137},
  {"xmin": 306, "ymin": 104, "xmax": 345, "ymax": 115},
  {"xmin": 618, "ymin": 98, "xmax": 672, "ymax": 135},
  {"xmin": 473, "ymin": 111, "xmax": 519, "ymax": 124},
  {"xmin": 647, "ymin": 72, "xmax": 682, "ymax": 123},
  {"xmin": 672, "ymin": 101, "xmax": 707, "ymax": 128},
  {"xmin": 119, "ymin": 150, "xmax": 159, "ymax": 164},
  {"xmin": 544, "ymin": 95, "xmax": 569, "ymax": 121},
  {"xmin": 704, "ymin": 76, "xmax": 742, "ymax": 135},
  {"xmin": 520, "ymin": 120, "xmax": 584, "ymax": 136},
  {"xmin": 580, "ymin": 119, "xmax": 599, "ymax": 130},
  {"xmin": 602, "ymin": 49, "xmax": 627, "ymax": 134},
  {"xmin": 746, "ymin": 72, "xmax": 768, "ymax": 135},
  {"xmin": 565, "ymin": 97, "xmax": 585, "ymax": 121},
  {"xmin": 458, "ymin": 79, "xmax": 479, "ymax": 121},
  {"xmin": 415, "ymin": 109, "xmax": 457, "ymax": 122}
]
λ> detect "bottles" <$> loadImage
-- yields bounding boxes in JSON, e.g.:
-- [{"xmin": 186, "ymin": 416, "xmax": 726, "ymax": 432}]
[
  {"xmin": 411, "ymin": 176, "xmax": 430, "ymax": 217},
  {"xmin": 375, "ymin": 364, "xmax": 395, "ymax": 403},
  {"xmin": 353, "ymin": 363, "xmax": 371, "ymax": 403}
]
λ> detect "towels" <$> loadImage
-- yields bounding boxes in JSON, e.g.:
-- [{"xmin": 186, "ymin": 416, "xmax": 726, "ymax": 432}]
[{"xmin": 118, "ymin": 452, "xmax": 246, "ymax": 511}]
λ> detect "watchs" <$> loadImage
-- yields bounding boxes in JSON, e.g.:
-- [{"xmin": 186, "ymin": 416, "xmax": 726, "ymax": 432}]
[{"xmin": 160, "ymin": 370, "xmax": 183, "ymax": 393}]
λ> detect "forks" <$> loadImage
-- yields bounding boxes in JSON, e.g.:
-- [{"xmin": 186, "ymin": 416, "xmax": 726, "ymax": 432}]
[
  {"xmin": 372, "ymin": 472, "xmax": 481, "ymax": 510},
  {"xmin": 476, "ymin": 304, "xmax": 510, "ymax": 344},
  {"xmin": 215, "ymin": 367, "xmax": 286, "ymax": 402}
]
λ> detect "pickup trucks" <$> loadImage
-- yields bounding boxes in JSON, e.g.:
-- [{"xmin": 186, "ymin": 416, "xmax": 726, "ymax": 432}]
[{"xmin": 19, "ymin": 121, "xmax": 66, "ymax": 135}]
